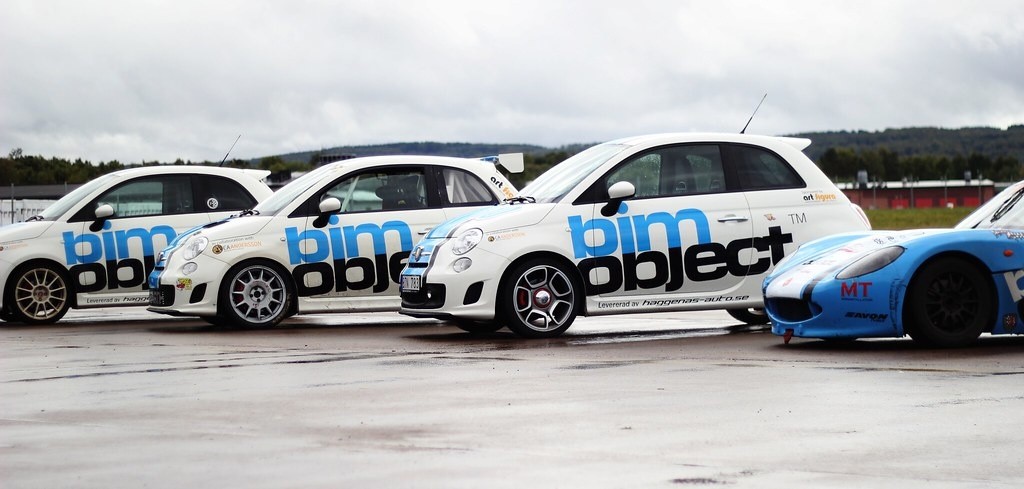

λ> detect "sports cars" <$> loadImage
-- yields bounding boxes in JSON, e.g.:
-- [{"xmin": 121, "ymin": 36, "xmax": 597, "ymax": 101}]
[{"xmin": 760, "ymin": 180, "xmax": 1023, "ymax": 348}]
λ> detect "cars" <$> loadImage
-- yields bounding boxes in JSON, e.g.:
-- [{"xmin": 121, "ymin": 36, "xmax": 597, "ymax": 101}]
[
  {"xmin": 145, "ymin": 152, "xmax": 527, "ymax": 334},
  {"xmin": 0, "ymin": 165, "xmax": 286, "ymax": 326},
  {"xmin": 398, "ymin": 135, "xmax": 873, "ymax": 341}
]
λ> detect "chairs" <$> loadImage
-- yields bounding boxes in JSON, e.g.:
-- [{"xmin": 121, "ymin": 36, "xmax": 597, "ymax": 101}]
[
  {"xmin": 660, "ymin": 153, "xmax": 739, "ymax": 193},
  {"xmin": 376, "ymin": 176, "xmax": 425, "ymax": 209}
]
